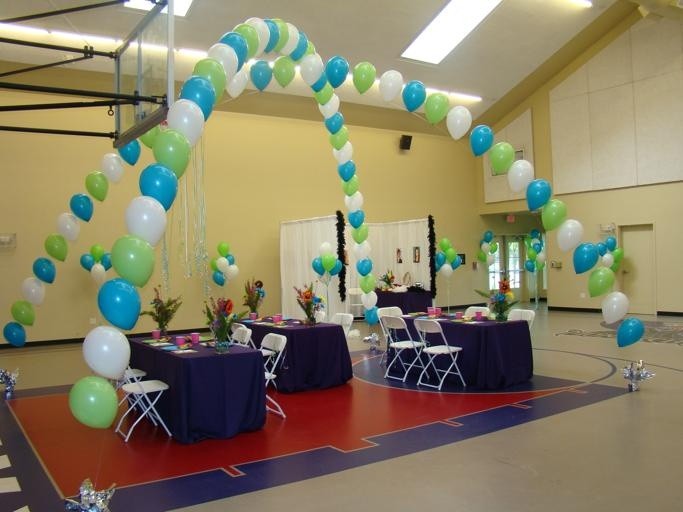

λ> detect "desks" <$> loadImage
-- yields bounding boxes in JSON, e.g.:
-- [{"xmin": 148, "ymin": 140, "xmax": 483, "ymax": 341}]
[
  {"xmin": 226, "ymin": 320, "xmax": 349, "ymax": 393},
  {"xmin": 375, "ymin": 286, "xmax": 431, "ymax": 314}
]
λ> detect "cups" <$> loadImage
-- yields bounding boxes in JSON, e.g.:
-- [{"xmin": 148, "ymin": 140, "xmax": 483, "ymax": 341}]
[
  {"xmin": 426, "ymin": 305, "xmax": 482, "ymax": 321},
  {"xmin": 248, "ymin": 312, "xmax": 282, "ymax": 325},
  {"xmin": 151, "ymin": 329, "xmax": 199, "ymax": 348}
]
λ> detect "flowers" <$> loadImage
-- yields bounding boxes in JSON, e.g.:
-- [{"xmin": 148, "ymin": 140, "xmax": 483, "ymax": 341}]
[
  {"xmin": 292, "ymin": 281, "xmax": 326, "ymax": 317},
  {"xmin": 242, "ymin": 278, "xmax": 266, "ymax": 312},
  {"xmin": 199, "ymin": 297, "xmax": 245, "ymax": 344},
  {"xmin": 473, "ymin": 279, "xmax": 515, "ymax": 317}
]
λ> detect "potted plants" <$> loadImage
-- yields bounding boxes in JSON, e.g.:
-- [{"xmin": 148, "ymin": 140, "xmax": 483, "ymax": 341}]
[{"xmin": 140, "ymin": 287, "xmax": 183, "ymax": 343}]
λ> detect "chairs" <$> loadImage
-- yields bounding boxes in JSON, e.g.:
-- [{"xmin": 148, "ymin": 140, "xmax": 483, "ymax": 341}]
[
  {"xmin": 347, "ymin": 287, "xmax": 367, "ymax": 320},
  {"xmin": 315, "ymin": 311, "xmax": 355, "ymax": 337},
  {"xmin": 376, "ymin": 305, "xmax": 535, "ymax": 390},
  {"xmin": 110, "ymin": 322, "xmax": 289, "ymax": 441}
]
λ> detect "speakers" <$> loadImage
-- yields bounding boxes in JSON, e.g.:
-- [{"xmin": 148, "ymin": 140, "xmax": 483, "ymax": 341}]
[{"xmin": 399, "ymin": 135, "xmax": 412, "ymax": 150}]
